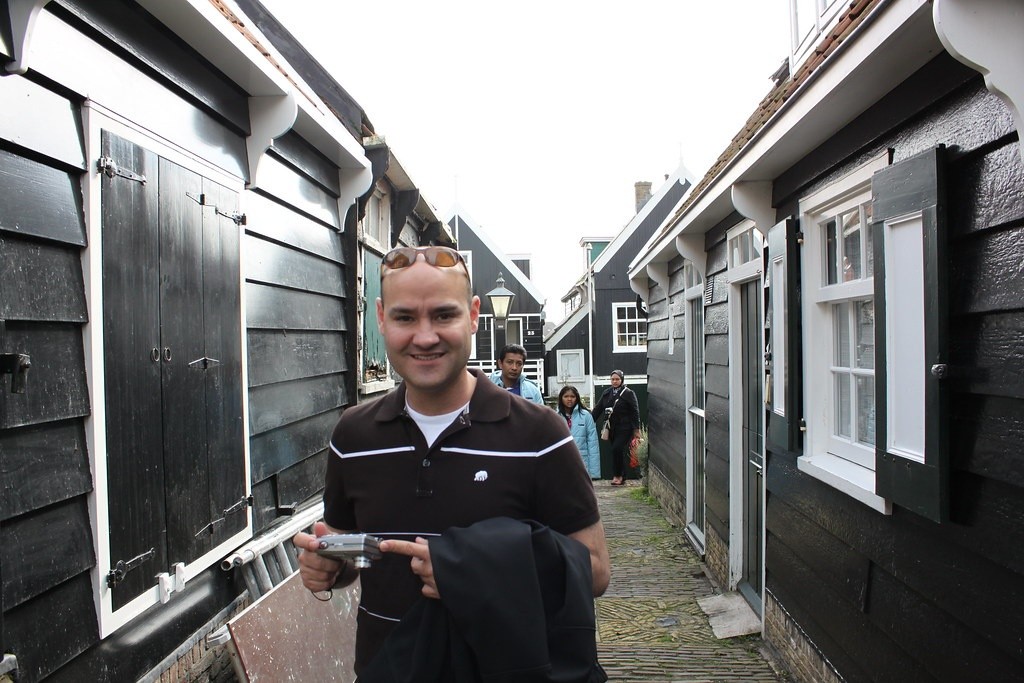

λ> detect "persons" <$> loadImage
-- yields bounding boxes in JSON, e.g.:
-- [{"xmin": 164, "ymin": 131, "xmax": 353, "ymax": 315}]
[
  {"xmin": 592, "ymin": 370, "xmax": 641, "ymax": 485},
  {"xmin": 293, "ymin": 246, "xmax": 610, "ymax": 683}
]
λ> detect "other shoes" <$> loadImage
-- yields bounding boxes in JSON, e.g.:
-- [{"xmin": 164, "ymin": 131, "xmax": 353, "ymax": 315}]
[{"xmin": 610, "ymin": 475, "xmax": 622, "ymax": 485}]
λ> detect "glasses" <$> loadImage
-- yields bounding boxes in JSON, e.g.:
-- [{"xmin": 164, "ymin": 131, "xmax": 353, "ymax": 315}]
[{"xmin": 378, "ymin": 247, "xmax": 471, "ymax": 311}]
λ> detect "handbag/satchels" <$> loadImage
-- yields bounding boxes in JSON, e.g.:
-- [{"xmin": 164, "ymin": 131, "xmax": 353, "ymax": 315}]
[
  {"xmin": 600, "ymin": 427, "xmax": 609, "ymax": 441},
  {"xmin": 629, "ymin": 435, "xmax": 645, "ymax": 468}
]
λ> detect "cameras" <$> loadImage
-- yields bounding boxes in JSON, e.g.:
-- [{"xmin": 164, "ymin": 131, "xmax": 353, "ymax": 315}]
[{"xmin": 316, "ymin": 533, "xmax": 384, "ymax": 570}]
